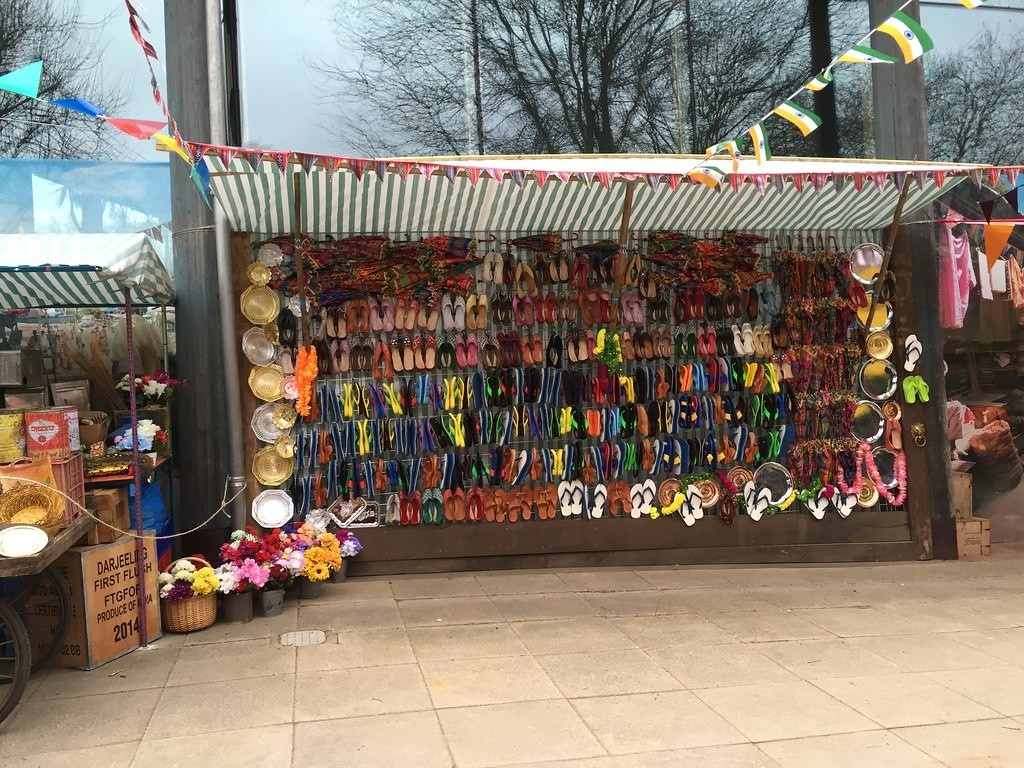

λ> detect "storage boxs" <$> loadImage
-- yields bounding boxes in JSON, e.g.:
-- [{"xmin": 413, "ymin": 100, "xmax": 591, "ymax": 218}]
[
  {"xmin": 27, "ymin": 531, "xmax": 164, "ymax": 673},
  {"xmin": 953, "ymin": 518, "xmax": 992, "ymax": 559},
  {"xmin": 26, "ymin": 405, "xmax": 80, "ymax": 456},
  {"xmin": 26, "ymin": 455, "xmax": 86, "ymax": 529},
  {"xmin": 948, "ymin": 465, "xmax": 974, "ymax": 516},
  {"xmin": 86, "ymin": 489, "xmax": 132, "ymax": 542},
  {"xmin": 78, "ymin": 411, "xmax": 108, "ymax": 445},
  {"xmin": 0, "ymin": 351, "xmax": 43, "ymax": 386}
]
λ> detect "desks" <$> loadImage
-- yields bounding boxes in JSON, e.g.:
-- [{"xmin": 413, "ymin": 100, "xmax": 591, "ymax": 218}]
[{"xmin": 82, "ymin": 455, "xmax": 177, "ymax": 530}]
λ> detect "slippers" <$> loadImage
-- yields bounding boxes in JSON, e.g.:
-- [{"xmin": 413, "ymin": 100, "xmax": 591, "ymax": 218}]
[
  {"xmin": 903, "ymin": 376, "xmax": 918, "ymax": 404},
  {"xmin": 295, "ymin": 354, "xmax": 796, "ymax": 525},
  {"xmin": 840, "ymin": 489, "xmax": 860, "ymax": 516},
  {"xmin": 831, "ymin": 487, "xmax": 847, "ymax": 518},
  {"xmin": 904, "ymin": 334, "xmax": 922, "ymax": 372},
  {"xmin": 915, "ymin": 375, "xmax": 930, "ymax": 403},
  {"xmin": 814, "ymin": 488, "xmax": 830, "ymax": 511},
  {"xmin": 801, "ymin": 489, "xmax": 825, "ymax": 520}
]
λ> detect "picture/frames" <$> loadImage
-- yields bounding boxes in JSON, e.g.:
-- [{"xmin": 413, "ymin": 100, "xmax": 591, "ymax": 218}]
[{"xmin": 52, "ymin": 380, "xmax": 91, "ymax": 411}]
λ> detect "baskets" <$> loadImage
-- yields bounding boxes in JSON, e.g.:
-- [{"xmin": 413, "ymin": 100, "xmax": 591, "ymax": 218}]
[
  {"xmin": 159, "ymin": 557, "xmax": 217, "ymax": 632},
  {"xmin": 0, "ymin": 484, "xmax": 66, "ymax": 537}
]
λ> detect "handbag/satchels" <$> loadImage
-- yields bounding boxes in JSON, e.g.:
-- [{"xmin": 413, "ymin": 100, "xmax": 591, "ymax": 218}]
[
  {"xmin": 0, "ymin": 457, "xmax": 69, "ymax": 529},
  {"xmin": 128, "ymin": 469, "xmax": 166, "ymax": 535}
]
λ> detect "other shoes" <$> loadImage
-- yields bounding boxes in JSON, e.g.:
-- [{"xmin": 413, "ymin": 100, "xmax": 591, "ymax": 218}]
[
  {"xmin": 886, "ymin": 418, "xmax": 903, "ymax": 450},
  {"xmin": 847, "ymin": 279, "xmax": 868, "ymax": 310},
  {"xmin": 871, "ymin": 270, "xmax": 897, "ymax": 303},
  {"xmin": 277, "ymin": 252, "xmax": 791, "ymax": 378}
]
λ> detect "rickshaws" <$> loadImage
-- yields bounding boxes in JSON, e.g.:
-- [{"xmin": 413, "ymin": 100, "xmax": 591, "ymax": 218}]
[{"xmin": 0, "ymin": 506, "xmax": 99, "ymax": 724}]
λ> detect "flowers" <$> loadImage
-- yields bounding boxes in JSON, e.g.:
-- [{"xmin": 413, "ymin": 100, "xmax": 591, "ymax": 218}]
[
  {"xmin": 116, "ymin": 369, "xmax": 189, "ymax": 401},
  {"xmin": 159, "ymin": 527, "xmax": 362, "ymax": 601},
  {"xmin": 114, "ymin": 419, "xmax": 169, "ymax": 454}
]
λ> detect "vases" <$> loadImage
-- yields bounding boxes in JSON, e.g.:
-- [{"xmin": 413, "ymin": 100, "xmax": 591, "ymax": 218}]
[
  {"xmin": 297, "ymin": 582, "xmax": 323, "ymax": 598},
  {"xmin": 331, "ymin": 561, "xmax": 348, "ymax": 582},
  {"xmin": 220, "ymin": 585, "xmax": 253, "ymax": 622},
  {"xmin": 259, "ymin": 588, "xmax": 286, "ymax": 616}
]
